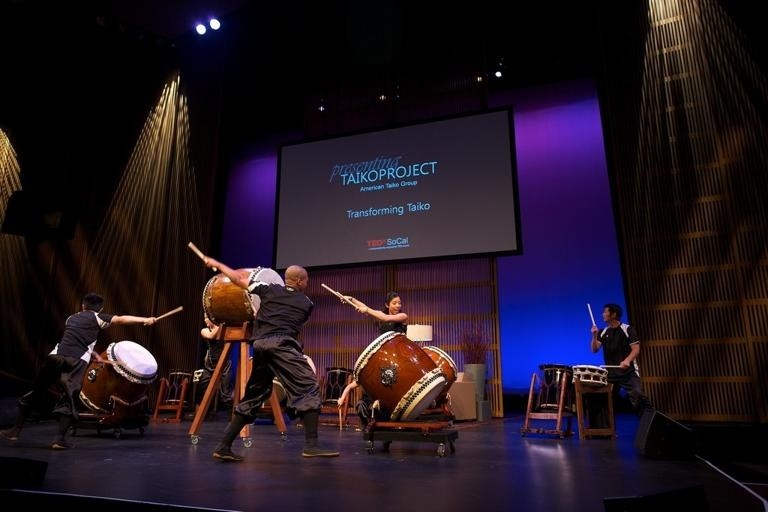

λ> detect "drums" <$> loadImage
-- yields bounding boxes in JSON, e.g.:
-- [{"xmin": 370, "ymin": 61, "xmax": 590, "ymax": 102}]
[
  {"xmin": 419, "ymin": 345, "xmax": 458, "ymax": 408},
  {"xmin": 572, "ymin": 365, "xmax": 609, "ymax": 385},
  {"xmin": 259, "ymin": 377, "xmax": 287, "ymax": 406},
  {"xmin": 353, "ymin": 330, "xmax": 447, "ymax": 423},
  {"xmin": 78, "ymin": 341, "xmax": 158, "ymax": 413},
  {"xmin": 167, "ymin": 368, "xmax": 194, "ymax": 388},
  {"xmin": 540, "ymin": 363, "xmax": 572, "ymax": 389},
  {"xmin": 322, "ymin": 366, "xmax": 354, "ymax": 406},
  {"xmin": 193, "ymin": 369, "xmax": 210, "ymax": 381},
  {"xmin": 202, "ymin": 267, "xmax": 286, "ymax": 327}
]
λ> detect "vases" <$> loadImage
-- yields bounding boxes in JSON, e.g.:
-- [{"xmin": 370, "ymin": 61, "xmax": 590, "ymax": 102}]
[{"xmin": 462, "ymin": 363, "xmax": 486, "ymax": 402}]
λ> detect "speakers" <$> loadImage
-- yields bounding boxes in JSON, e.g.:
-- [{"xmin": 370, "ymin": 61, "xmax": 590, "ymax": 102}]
[{"xmin": 631, "ymin": 409, "xmax": 694, "ymax": 463}]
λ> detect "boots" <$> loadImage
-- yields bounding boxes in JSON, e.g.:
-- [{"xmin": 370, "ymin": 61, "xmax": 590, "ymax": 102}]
[
  {"xmin": 52, "ymin": 415, "xmax": 68, "ymax": 449},
  {"xmin": 213, "ymin": 414, "xmax": 248, "ymax": 460},
  {"xmin": 301, "ymin": 411, "xmax": 339, "ymax": 456},
  {"xmin": 0, "ymin": 416, "xmax": 24, "ymax": 440}
]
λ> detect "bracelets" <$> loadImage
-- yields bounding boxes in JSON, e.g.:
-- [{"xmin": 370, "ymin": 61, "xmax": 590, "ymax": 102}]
[{"xmin": 348, "ymin": 296, "xmax": 353, "ymax": 302}]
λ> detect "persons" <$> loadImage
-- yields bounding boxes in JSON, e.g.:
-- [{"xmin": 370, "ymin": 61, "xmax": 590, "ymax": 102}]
[
  {"xmin": 338, "ymin": 282, "xmax": 409, "ymax": 454},
  {"xmin": 590, "ymin": 304, "xmax": 652, "ymax": 419},
  {"xmin": 1, "ymin": 292, "xmax": 156, "ymax": 452},
  {"xmin": 201, "ymin": 258, "xmax": 341, "ymax": 463}
]
